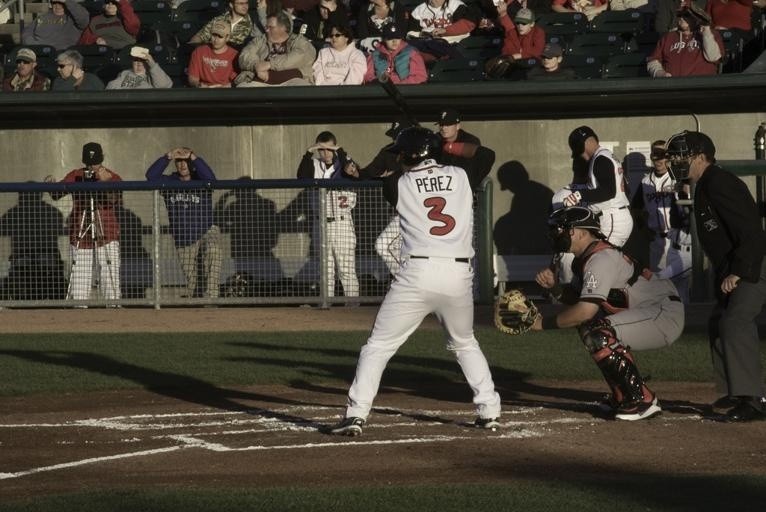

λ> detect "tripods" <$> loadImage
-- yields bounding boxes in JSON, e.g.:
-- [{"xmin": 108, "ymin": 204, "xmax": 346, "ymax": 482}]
[{"xmin": 64, "ymin": 195, "xmax": 121, "ymax": 308}]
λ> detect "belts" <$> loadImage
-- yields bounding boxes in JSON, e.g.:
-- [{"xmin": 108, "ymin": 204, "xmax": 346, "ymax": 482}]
[
  {"xmin": 410, "ymin": 255, "xmax": 469, "ymax": 262},
  {"xmin": 326, "ymin": 216, "xmax": 344, "ymax": 222},
  {"xmin": 650, "ymin": 230, "xmax": 668, "ymax": 237},
  {"xmin": 673, "ymin": 242, "xmax": 690, "ymax": 251}
]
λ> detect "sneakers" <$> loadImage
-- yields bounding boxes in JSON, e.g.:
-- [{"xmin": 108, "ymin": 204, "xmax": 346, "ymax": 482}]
[
  {"xmin": 328, "ymin": 417, "xmax": 365, "ymax": 437},
  {"xmin": 601, "ymin": 391, "xmax": 629, "ymax": 411},
  {"xmin": 475, "ymin": 417, "xmax": 502, "ymax": 429},
  {"xmin": 614, "ymin": 392, "xmax": 663, "ymax": 422}
]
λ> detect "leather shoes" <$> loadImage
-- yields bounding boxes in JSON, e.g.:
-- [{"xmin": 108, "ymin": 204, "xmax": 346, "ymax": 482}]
[
  {"xmin": 712, "ymin": 395, "xmax": 752, "ymax": 415},
  {"xmin": 721, "ymin": 399, "xmax": 766, "ymax": 423}
]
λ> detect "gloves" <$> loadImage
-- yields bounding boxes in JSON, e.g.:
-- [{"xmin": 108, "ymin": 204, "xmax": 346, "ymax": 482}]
[{"xmin": 563, "ymin": 191, "xmax": 583, "ymax": 206}]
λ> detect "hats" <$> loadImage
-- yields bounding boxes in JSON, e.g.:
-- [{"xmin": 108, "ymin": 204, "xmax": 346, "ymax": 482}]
[
  {"xmin": 512, "ymin": 8, "xmax": 536, "ymax": 23},
  {"xmin": 82, "ymin": 142, "xmax": 102, "ymax": 165},
  {"xmin": 539, "ymin": 43, "xmax": 562, "ymax": 57},
  {"xmin": 382, "ymin": 23, "xmax": 405, "ymax": 39},
  {"xmin": 212, "ymin": 21, "xmax": 231, "ymax": 37},
  {"xmin": 14, "ymin": 49, "xmax": 36, "ymax": 64},
  {"xmin": 385, "ymin": 120, "xmax": 417, "ymax": 138},
  {"xmin": 676, "ymin": 1, "xmax": 691, "ymax": 10},
  {"xmin": 435, "ymin": 108, "xmax": 461, "ymax": 127},
  {"xmin": 570, "ymin": 126, "xmax": 595, "ymax": 158}
]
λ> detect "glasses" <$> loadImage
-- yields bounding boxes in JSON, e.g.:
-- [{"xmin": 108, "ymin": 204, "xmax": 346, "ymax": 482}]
[
  {"xmin": 15, "ymin": 60, "xmax": 30, "ymax": 64},
  {"xmin": 234, "ymin": 1, "xmax": 250, "ymax": 6},
  {"xmin": 516, "ymin": 21, "xmax": 527, "ymax": 25},
  {"xmin": 329, "ymin": 32, "xmax": 345, "ymax": 38},
  {"xmin": 676, "ymin": 14, "xmax": 691, "ymax": 18},
  {"xmin": 263, "ymin": 24, "xmax": 279, "ymax": 31},
  {"xmin": 56, "ymin": 63, "xmax": 75, "ymax": 70},
  {"xmin": 541, "ymin": 54, "xmax": 552, "ymax": 59}
]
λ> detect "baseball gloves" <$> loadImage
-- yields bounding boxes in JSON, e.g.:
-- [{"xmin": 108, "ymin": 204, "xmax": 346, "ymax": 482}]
[{"xmin": 494, "ymin": 290, "xmax": 539, "ymax": 334}]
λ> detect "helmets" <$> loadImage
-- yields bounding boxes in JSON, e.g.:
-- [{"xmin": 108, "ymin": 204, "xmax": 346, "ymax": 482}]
[
  {"xmin": 545, "ymin": 206, "xmax": 602, "ymax": 268},
  {"xmin": 395, "ymin": 126, "xmax": 444, "ymax": 168},
  {"xmin": 665, "ymin": 129, "xmax": 717, "ymax": 193}
]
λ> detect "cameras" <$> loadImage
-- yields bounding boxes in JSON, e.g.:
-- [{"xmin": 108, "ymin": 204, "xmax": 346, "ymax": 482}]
[
  {"xmin": 75, "ymin": 169, "xmax": 95, "ymax": 183},
  {"xmin": 130, "ymin": 46, "xmax": 149, "ymax": 59}
]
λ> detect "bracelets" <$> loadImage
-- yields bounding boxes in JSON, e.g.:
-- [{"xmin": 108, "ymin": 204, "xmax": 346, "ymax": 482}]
[
  {"xmin": 549, "ymin": 280, "xmax": 562, "ymax": 298},
  {"xmin": 188, "ymin": 151, "xmax": 193, "ymax": 159},
  {"xmin": 542, "ymin": 316, "xmax": 558, "ymax": 330},
  {"xmin": 444, "ymin": 142, "xmax": 464, "ymax": 157}
]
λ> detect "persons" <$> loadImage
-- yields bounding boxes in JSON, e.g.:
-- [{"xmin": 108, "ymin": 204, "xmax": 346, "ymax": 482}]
[
  {"xmin": 44, "ymin": 142, "xmax": 122, "ymax": 308},
  {"xmin": 530, "ymin": 206, "xmax": 685, "ymax": 422},
  {"xmin": 434, "ymin": 110, "xmax": 480, "ymax": 167},
  {"xmin": 1, "ymin": 0, "xmax": 765, "ymax": 91},
  {"xmin": 558, "ymin": 126, "xmax": 633, "ymax": 285},
  {"xmin": 663, "ymin": 130, "xmax": 766, "ymax": 421},
  {"xmin": 297, "ymin": 131, "xmax": 360, "ymax": 307},
  {"xmin": 633, "ymin": 140, "xmax": 693, "ymax": 283},
  {"xmin": 365, "ymin": 120, "xmax": 411, "ymax": 177},
  {"xmin": 146, "ymin": 147, "xmax": 222, "ymax": 307},
  {"xmin": 320, "ymin": 127, "xmax": 502, "ymax": 436}
]
